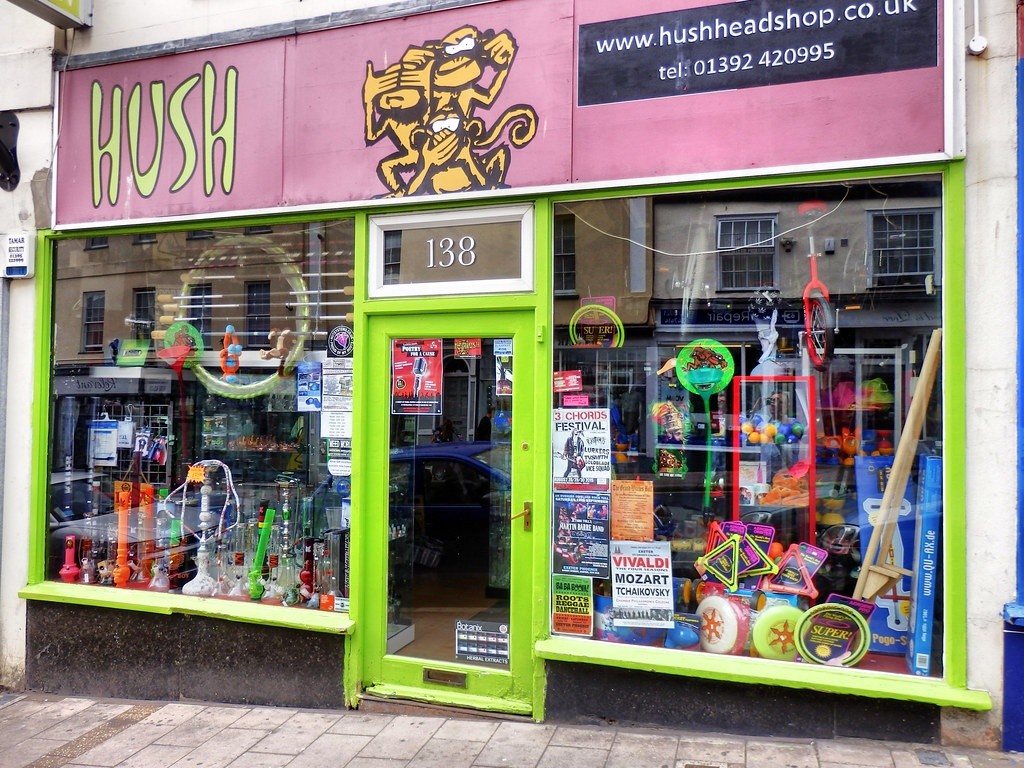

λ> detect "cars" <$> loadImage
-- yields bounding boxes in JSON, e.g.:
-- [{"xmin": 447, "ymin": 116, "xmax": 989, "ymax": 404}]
[
  {"xmin": 297, "ymin": 440, "xmax": 676, "ymax": 568},
  {"xmin": 48, "ymin": 471, "xmax": 229, "ymax": 587}
]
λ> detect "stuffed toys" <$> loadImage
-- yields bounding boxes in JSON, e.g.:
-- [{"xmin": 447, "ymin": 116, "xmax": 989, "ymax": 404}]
[
  {"xmin": 260, "ymin": 327, "xmax": 297, "ymax": 378},
  {"xmin": 218, "ymin": 324, "xmax": 243, "ymax": 386}
]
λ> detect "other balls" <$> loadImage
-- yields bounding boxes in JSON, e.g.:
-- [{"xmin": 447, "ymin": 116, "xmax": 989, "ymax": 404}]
[{"xmin": 739, "ymin": 415, "xmax": 804, "ymax": 444}]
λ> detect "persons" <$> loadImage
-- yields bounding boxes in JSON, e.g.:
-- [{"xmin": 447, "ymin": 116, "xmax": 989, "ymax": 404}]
[{"xmin": 561, "ymin": 429, "xmax": 585, "ymax": 482}]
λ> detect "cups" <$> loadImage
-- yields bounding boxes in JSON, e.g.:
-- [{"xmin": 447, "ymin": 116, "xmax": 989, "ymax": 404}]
[{"xmin": 325, "ymin": 507, "xmax": 346, "ymax": 529}]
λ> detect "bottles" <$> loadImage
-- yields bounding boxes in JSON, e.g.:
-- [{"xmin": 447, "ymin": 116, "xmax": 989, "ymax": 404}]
[{"xmin": 243, "ymin": 420, "xmax": 253, "ymax": 436}]
